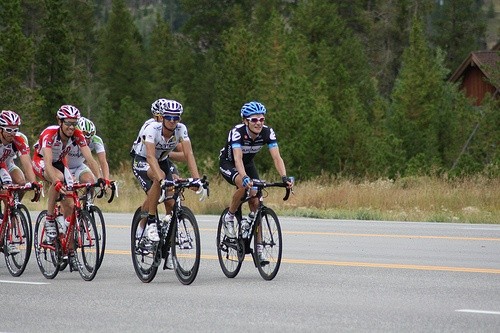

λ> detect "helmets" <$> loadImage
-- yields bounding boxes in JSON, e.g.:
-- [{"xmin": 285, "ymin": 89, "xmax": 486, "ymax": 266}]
[
  {"xmin": 0, "ymin": 110, "xmax": 21, "ymax": 126},
  {"xmin": 78, "ymin": 117, "xmax": 96, "ymax": 136},
  {"xmin": 241, "ymin": 102, "xmax": 266, "ymax": 118},
  {"xmin": 150, "ymin": 99, "xmax": 169, "ymax": 116},
  {"xmin": 159, "ymin": 100, "xmax": 184, "ymax": 116},
  {"xmin": 57, "ymin": 105, "xmax": 80, "ymax": 120}
]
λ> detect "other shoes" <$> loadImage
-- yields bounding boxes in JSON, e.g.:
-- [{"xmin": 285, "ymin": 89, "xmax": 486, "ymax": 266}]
[{"xmin": 137, "ymin": 221, "xmax": 148, "ymax": 241}]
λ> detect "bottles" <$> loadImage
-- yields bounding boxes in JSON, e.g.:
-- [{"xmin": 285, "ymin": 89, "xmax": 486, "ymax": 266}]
[
  {"xmin": 159, "ymin": 220, "xmax": 167, "ymax": 239},
  {"xmin": 0, "ymin": 214, "xmax": 4, "ymax": 226},
  {"xmin": 55, "ymin": 218, "xmax": 65, "ymax": 234},
  {"xmin": 64, "ymin": 216, "xmax": 71, "ymax": 234},
  {"xmin": 240, "ymin": 218, "xmax": 248, "ymax": 239},
  {"xmin": 163, "ymin": 213, "xmax": 173, "ymax": 233},
  {"xmin": 245, "ymin": 212, "xmax": 254, "ymax": 230}
]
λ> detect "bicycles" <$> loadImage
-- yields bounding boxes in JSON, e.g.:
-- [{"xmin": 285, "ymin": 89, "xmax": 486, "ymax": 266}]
[
  {"xmin": 0, "ymin": 177, "xmax": 118, "ymax": 282},
  {"xmin": 216, "ymin": 176, "xmax": 297, "ymax": 280},
  {"xmin": 130, "ymin": 176, "xmax": 211, "ymax": 286}
]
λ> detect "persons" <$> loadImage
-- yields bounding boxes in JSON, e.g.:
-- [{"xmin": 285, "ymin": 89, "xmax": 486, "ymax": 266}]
[
  {"xmin": 131, "ymin": 100, "xmax": 206, "ymax": 270},
  {"xmin": 218, "ymin": 99, "xmax": 296, "ymax": 266},
  {"xmin": 30, "ymin": 101, "xmax": 108, "ymax": 270},
  {"xmin": 58, "ymin": 116, "xmax": 112, "ymax": 238},
  {"xmin": 0, "ymin": 110, "xmax": 44, "ymax": 257},
  {"xmin": 128, "ymin": 98, "xmax": 190, "ymax": 234}
]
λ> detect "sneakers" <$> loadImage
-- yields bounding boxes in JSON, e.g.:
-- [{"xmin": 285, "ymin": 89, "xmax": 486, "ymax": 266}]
[
  {"xmin": 256, "ymin": 249, "xmax": 269, "ymax": 266},
  {"xmin": 147, "ymin": 223, "xmax": 160, "ymax": 241},
  {"xmin": 222, "ymin": 215, "xmax": 237, "ymax": 238},
  {"xmin": 167, "ymin": 254, "xmax": 174, "ymax": 269},
  {"xmin": 7, "ymin": 243, "xmax": 20, "ymax": 254},
  {"xmin": 45, "ymin": 220, "xmax": 57, "ymax": 238},
  {"xmin": 71, "ymin": 256, "xmax": 82, "ymax": 271}
]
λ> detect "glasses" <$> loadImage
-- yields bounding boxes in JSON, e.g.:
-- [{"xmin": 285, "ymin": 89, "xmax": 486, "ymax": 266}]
[
  {"xmin": 63, "ymin": 121, "xmax": 78, "ymax": 126},
  {"xmin": 165, "ymin": 116, "xmax": 180, "ymax": 121},
  {"xmin": 0, "ymin": 127, "xmax": 19, "ymax": 133},
  {"xmin": 245, "ymin": 117, "xmax": 265, "ymax": 122}
]
